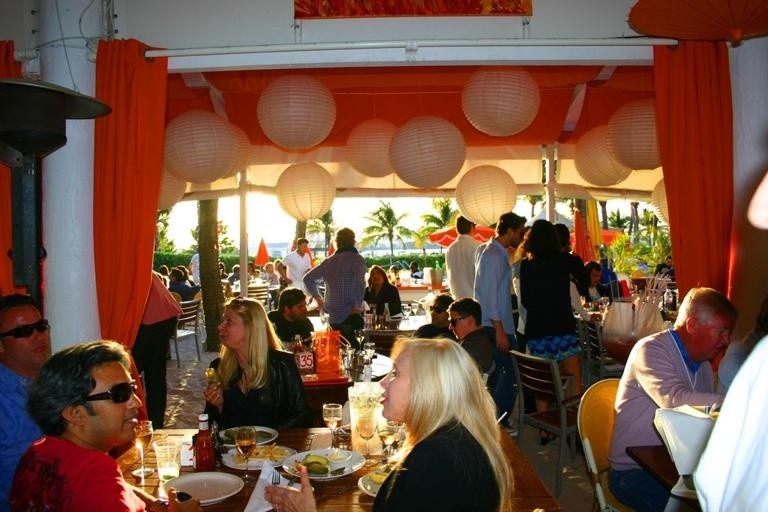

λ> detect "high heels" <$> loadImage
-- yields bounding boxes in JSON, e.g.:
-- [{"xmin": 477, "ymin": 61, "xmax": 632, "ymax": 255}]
[{"xmin": 540, "ymin": 429, "xmax": 551, "ymax": 445}]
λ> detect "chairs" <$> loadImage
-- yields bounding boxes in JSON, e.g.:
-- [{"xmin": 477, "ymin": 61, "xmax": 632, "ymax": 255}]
[
  {"xmin": 246, "ymin": 286, "xmax": 270, "ymax": 313},
  {"xmin": 584, "ymin": 319, "xmax": 623, "ymax": 377},
  {"xmin": 364, "ymin": 328, "xmax": 416, "ymax": 359},
  {"xmin": 508, "ymin": 351, "xmax": 579, "ymax": 503},
  {"xmin": 576, "ymin": 379, "xmax": 633, "ymax": 512},
  {"xmin": 172, "ymin": 300, "xmax": 202, "ymax": 368}
]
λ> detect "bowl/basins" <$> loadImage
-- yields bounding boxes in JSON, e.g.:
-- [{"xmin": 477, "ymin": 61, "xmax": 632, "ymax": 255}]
[{"xmin": 148, "ymin": 432, "xmax": 168, "ymax": 449}]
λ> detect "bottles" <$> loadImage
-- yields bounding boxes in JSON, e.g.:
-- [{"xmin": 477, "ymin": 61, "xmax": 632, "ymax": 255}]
[
  {"xmin": 435, "ymin": 261, "xmax": 441, "ymax": 270},
  {"xmin": 292, "ymin": 334, "xmax": 303, "ymax": 353},
  {"xmin": 365, "ymin": 311, "xmax": 373, "ymax": 329},
  {"xmin": 411, "ymin": 304, "xmax": 418, "ymax": 317},
  {"xmin": 212, "ymin": 425, "xmax": 224, "ymax": 468},
  {"xmin": 322, "ymin": 313, "xmax": 333, "ymax": 331},
  {"xmin": 347, "ymin": 348, "xmax": 355, "ymax": 369},
  {"xmin": 193, "ymin": 414, "xmax": 216, "ymax": 472},
  {"xmin": 663, "ymin": 289, "xmax": 673, "ymax": 312},
  {"xmin": 370, "ymin": 304, "xmax": 377, "ymax": 320},
  {"xmin": 383, "ymin": 303, "xmax": 391, "ymax": 329}
]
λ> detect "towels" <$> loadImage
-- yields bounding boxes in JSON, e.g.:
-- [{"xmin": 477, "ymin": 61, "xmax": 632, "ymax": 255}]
[
  {"xmin": 243, "ymin": 460, "xmax": 316, "ymax": 512},
  {"xmin": 653, "ymin": 405, "xmax": 714, "ymax": 476}
]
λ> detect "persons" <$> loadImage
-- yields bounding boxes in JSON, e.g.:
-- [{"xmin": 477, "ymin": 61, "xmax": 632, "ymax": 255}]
[
  {"xmin": 9, "ymin": 339, "xmax": 208, "ymax": 510},
  {"xmin": 132, "ymin": 211, "xmax": 403, "ymax": 426},
  {"xmin": 0, "ymin": 293, "xmax": 50, "ymax": 512},
  {"xmin": 608, "ymin": 287, "xmax": 740, "ymax": 511},
  {"xmin": 413, "ymin": 212, "xmax": 679, "ymax": 446},
  {"xmin": 717, "ymin": 294, "xmax": 768, "ymax": 397},
  {"xmin": 264, "ymin": 339, "xmax": 514, "ymax": 511}
]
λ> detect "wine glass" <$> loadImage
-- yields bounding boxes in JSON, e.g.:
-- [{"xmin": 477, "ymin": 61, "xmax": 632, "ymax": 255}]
[
  {"xmin": 132, "ymin": 421, "xmax": 154, "ymax": 477},
  {"xmin": 356, "ymin": 417, "xmax": 379, "ymax": 467},
  {"xmin": 323, "ymin": 404, "xmax": 343, "ymax": 450},
  {"xmin": 580, "ymin": 297, "xmax": 586, "ymax": 306},
  {"xmin": 378, "ymin": 427, "xmax": 395, "ymax": 467},
  {"xmin": 364, "ymin": 342, "xmax": 375, "ymax": 372},
  {"xmin": 401, "ymin": 304, "xmax": 408, "ymax": 316},
  {"xmin": 234, "ymin": 427, "xmax": 259, "ymax": 483},
  {"xmin": 402, "ymin": 307, "xmax": 411, "ymax": 319},
  {"xmin": 602, "ymin": 297, "xmax": 609, "ymax": 312}
]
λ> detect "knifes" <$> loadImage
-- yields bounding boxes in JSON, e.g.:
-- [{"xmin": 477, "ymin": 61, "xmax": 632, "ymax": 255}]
[{"xmin": 288, "ymin": 476, "xmax": 297, "ymax": 486}]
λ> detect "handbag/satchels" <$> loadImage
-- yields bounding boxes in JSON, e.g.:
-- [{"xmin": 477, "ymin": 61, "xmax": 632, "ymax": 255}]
[{"xmin": 312, "ymin": 330, "xmax": 351, "ymax": 374}]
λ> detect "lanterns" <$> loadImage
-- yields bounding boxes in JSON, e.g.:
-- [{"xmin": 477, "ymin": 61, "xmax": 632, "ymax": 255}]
[{"xmin": 157, "ymin": 66, "xmax": 670, "ymax": 226}]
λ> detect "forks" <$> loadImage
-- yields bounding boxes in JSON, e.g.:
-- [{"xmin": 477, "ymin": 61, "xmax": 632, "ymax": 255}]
[{"xmin": 273, "ymin": 471, "xmax": 280, "ymax": 485}]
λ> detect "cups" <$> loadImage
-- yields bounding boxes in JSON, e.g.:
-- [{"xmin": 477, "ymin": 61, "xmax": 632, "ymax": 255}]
[{"xmin": 152, "ymin": 439, "xmax": 182, "ymax": 481}]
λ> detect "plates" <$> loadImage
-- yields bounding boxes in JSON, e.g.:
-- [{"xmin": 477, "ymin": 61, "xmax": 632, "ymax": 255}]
[
  {"xmin": 218, "ymin": 425, "xmax": 279, "ymax": 446},
  {"xmin": 222, "ymin": 446, "xmax": 298, "ymax": 470},
  {"xmin": 163, "ymin": 472, "xmax": 244, "ymax": 506},
  {"xmin": 282, "ymin": 449, "xmax": 366, "ymax": 482},
  {"xmin": 357, "ymin": 468, "xmax": 407, "ymax": 497}
]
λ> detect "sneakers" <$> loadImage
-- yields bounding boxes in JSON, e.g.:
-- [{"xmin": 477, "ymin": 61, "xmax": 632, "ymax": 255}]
[{"xmin": 504, "ymin": 425, "xmax": 519, "ymax": 438}]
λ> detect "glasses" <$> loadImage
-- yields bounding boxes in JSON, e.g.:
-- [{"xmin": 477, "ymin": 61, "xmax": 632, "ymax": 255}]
[
  {"xmin": 447, "ymin": 317, "xmax": 467, "ymax": 325},
  {"xmin": 428, "ymin": 304, "xmax": 446, "ymax": 314},
  {"xmin": 1, "ymin": 319, "xmax": 49, "ymax": 338},
  {"xmin": 86, "ymin": 381, "xmax": 139, "ymax": 403},
  {"xmin": 233, "ymin": 296, "xmax": 253, "ymax": 321},
  {"xmin": 515, "ymin": 230, "xmax": 525, "ymax": 239}
]
